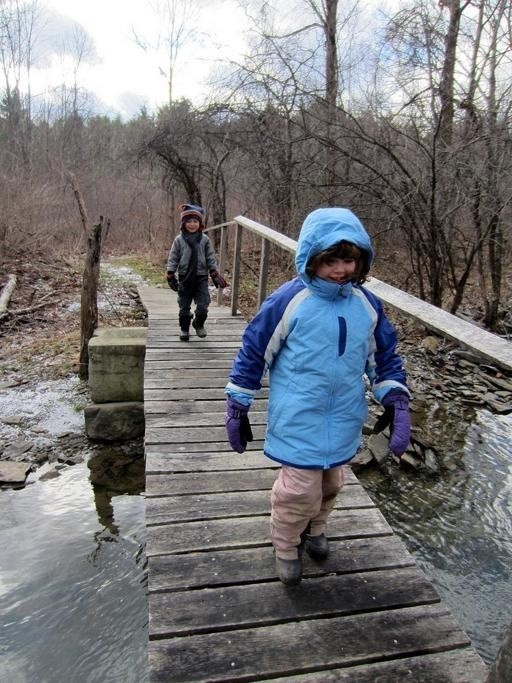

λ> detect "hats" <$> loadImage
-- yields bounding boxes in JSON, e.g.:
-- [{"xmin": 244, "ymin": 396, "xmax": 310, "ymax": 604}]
[{"xmin": 181, "ymin": 204, "xmax": 204, "ymax": 231}]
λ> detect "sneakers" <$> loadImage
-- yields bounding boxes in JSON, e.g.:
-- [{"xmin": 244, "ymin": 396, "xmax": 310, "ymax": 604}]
[
  {"xmin": 180, "ymin": 329, "xmax": 189, "ymax": 341},
  {"xmin": 275, "ymin": 553, "xmax": 303, "ymax": 587},
  {"xmin": 192, "ymin": 320, "xmax": 207, "ymax": 337},
  {"xmin": 305, "ymin": 535, "xmax": 330, "ymax": 561}
]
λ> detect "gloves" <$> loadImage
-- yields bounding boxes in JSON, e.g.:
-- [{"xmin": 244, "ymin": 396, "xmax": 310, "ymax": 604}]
[
  {"xmin": 372, "ymin": 392, "xmax": 411, "ymax": 456},
  {"xmin": 210, "ymin": 270, "xmax": 227, "ymax": 288},
  {"xmin": 167, "ymin": 274, "xmax": 178, "ymax": 291},
  {"xmin": 224, "ymin": 397, "xmax": 253, "ymax": 453}
]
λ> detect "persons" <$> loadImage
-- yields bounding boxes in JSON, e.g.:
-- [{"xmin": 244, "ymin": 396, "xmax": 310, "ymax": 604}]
[
  {"xmin": 223, "ymin": 206, "xmax": 412, "ymax": 585},
  {"xmin": 165, "ymin": 203, "xmax": 227, "ymax": 341}
]
